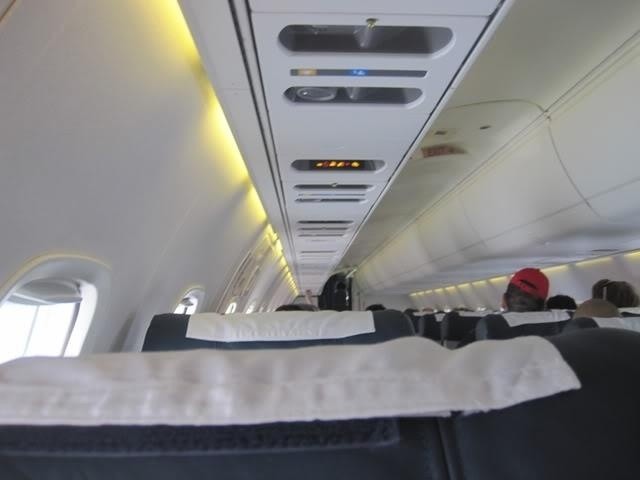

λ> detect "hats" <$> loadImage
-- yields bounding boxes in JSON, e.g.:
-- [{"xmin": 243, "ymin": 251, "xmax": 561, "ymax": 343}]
[{"xmin": 511, "ymin": 268, "xmax": 549, "ymax": 299}]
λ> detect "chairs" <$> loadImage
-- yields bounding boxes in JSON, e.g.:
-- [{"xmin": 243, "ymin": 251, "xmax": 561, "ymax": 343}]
[{"xmin": 1, "ymin": 302, "xmax": 640, "ymax": 480}]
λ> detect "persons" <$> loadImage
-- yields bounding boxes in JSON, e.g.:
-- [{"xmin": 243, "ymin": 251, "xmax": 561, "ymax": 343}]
[{"xmin": 499, "ymin": 268, "xmax": 640, "ymax": 318}]
